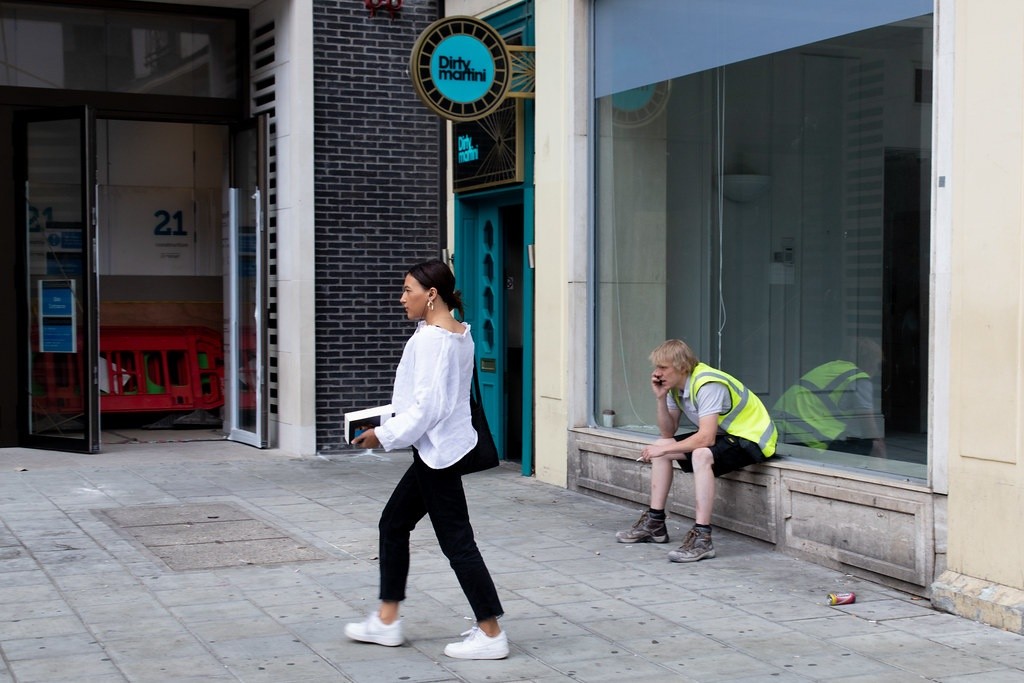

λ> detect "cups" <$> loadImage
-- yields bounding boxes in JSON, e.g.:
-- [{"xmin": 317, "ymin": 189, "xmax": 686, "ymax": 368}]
[{"xmin": 602, "ymin": 410, "xmax": 615, "ymax": 428}]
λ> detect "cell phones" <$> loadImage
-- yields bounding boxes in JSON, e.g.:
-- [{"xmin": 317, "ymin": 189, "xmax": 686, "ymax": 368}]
[{"xmin": 655, "ymin": 376, "xmax": 662, "ymax": 386}]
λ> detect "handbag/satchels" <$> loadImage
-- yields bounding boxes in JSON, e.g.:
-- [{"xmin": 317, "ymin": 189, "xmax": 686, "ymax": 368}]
[{"xmin": 457, "ymin": 353, "xmax": 500, "ymax": 476}]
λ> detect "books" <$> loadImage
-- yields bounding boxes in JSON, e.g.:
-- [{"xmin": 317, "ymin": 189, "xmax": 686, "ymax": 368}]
[{"xmin": 344, "ymin": 404, "xmax": 395, "ymax": 445}]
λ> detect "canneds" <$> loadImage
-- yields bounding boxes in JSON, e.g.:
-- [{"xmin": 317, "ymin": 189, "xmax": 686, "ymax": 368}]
[{"xmin": 826, "ymin": 592, "xmax": 856, "ymax": 606}]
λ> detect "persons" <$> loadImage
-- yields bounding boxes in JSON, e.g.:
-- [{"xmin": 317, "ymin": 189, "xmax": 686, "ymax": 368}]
[
  {"xmin": 769, "ymin": 339, "xmax": 888, "ymax": 459},
  {"xmin": 343, "ymin": 259, "xmax": 510, "ymax": 660},
  {"xmin": 614, "ymin": 339, "xmax": 779, "ymax": 563}
]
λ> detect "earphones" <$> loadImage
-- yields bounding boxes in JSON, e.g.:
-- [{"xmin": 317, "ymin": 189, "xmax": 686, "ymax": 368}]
[{"xmin": 428, "ymin": 293, "xmax": 433, "ymax": 297}]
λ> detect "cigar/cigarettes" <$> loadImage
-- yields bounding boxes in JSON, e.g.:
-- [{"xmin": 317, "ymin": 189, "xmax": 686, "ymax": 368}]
[{"xmin": 636, "ymin": 456, "xmax": 643, "ymax": 461}]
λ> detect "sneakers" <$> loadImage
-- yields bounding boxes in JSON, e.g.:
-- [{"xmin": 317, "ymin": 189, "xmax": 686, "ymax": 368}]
[
  {"xmin": 444, "ymin": 628, "xmax": 511, "ymax": 661},
  {"xmin": 344, "ymin": 612, "xmax": 405, "ymax": 648},
  {"xmin": 666, "ymin": 527, "xmax": 716, "ymax": 563},
  {"xmin": 615, "ymin": 511, "xmax": 669, "ymax": 543}
]
